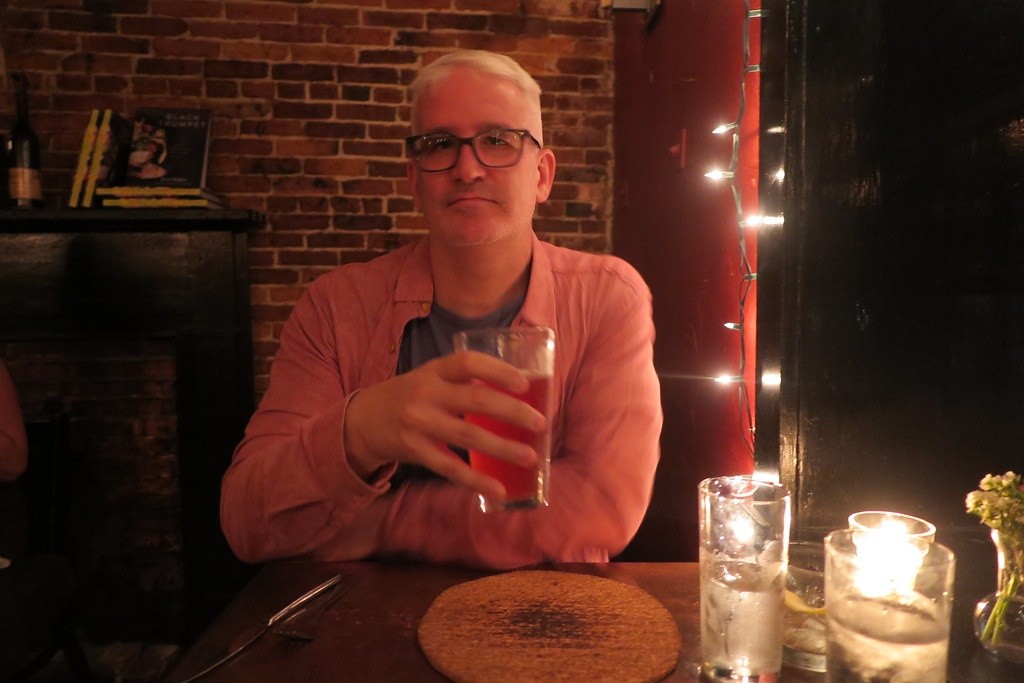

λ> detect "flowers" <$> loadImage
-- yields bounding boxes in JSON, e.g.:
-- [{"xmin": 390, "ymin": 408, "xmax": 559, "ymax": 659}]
[{"xmin": 964, "ymin": 472, "xmax": 1024, "ymax": 647}]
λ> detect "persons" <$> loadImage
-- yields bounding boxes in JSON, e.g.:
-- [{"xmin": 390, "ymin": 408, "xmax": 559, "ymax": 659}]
[{"xmin": 221, "ymin": 51, "xmax": 663, "ymax": 568}]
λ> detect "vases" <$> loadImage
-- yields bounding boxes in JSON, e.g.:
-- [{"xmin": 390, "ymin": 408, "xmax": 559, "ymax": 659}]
[{"xmin": 972, "ymin": 528, "xmax": 1024, "ymax": 665}]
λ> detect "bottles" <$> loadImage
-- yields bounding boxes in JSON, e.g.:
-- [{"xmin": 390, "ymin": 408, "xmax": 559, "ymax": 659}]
[{"xmin": 6, "ymin": 71, "xmax": 40, "ymax": 207}]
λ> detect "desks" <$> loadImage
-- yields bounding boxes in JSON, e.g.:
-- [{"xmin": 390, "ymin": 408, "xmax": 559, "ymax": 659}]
[
  {"xmin": 0, "ymin": 202, "xmax": 264, "ymax": 645},
  {"xmin": 151, "ymin": 563, "xmax": 1024, "ymax": 683}
]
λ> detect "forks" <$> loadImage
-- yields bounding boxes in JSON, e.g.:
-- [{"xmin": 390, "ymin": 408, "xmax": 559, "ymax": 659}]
[{"xmin": 271, "ymin": 568, "xmax": 355, "ymax": 641}]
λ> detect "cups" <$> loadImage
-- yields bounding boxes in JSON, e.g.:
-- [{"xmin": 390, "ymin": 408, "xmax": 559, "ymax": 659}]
[
  {"xmin": 782, "ymin": 542, "xmax": 871, "ymax": 675},
  {"xmin": 849, "ymin": 511, "xmax": 936, "ymax": 543},
  {"xmin": 825, "ymin": 524, "xmax": 957, "ymax": 683},
  {"xmin": 698, "ymin": 476, "xmax": 790, "ymax": 683},
  {"xmin": 456, "ymin": 325, "xmax": 557, "ymax": 509}
]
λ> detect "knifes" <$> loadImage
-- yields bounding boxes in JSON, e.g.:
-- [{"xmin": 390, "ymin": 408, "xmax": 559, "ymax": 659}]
[{"xmin": 177, "ymin": 572, "xmax": 343, "ymax": 683}]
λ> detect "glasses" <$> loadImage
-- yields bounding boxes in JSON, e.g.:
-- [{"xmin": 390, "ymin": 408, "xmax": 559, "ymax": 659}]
[{"xmin": 404, "ymin": 128, "xmax": 541, "ymax": 172}]
